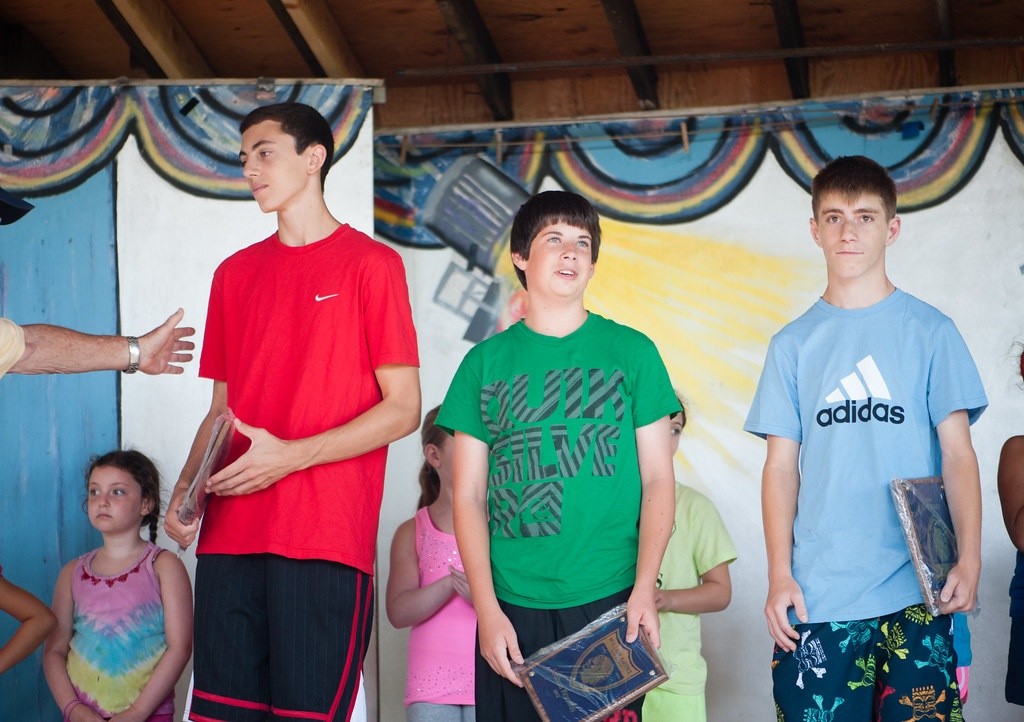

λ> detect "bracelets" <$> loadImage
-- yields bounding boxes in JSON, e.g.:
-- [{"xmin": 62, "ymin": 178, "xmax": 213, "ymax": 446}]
[
  {"xmin": 123, "ymin": 336, "xmax": 140, "ymax": 374},
  {"xmin": 61, "ymin": 698, "xmax": 82, "ymax": 720}
]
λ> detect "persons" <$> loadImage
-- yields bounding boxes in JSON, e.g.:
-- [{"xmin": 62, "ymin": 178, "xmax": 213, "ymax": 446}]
[
  {"xmin": 162, "ymin": 103, "xmax": 422, "ymax": 722},
  {"xmin": 0, "ymin": 565, "xmax": 58, "ymax": 675},
  {"xmin": 43, "ymin": 450, "xmax": 193, "ymax": 722},
  {"xmin": 997, "ymin": 341, "xmax": 1024, "ymax": 706},
  {"xmin": 385, "ymin": 404, "xmax": 477, "ymax": 722},
  {"xmin": 642, "ymin": 397, "xmax": 738, "ymax": 722},
  {"xmin": 430, "ymin": 191, "xmax": 683, "ymax": 722},
  {"xmin": 743, "ymin": 156, "xmax": 989, "ymax": 722},
  {"xmin": 1, "ymin": 308, "xmax": 195, "ymax": 379}
]
